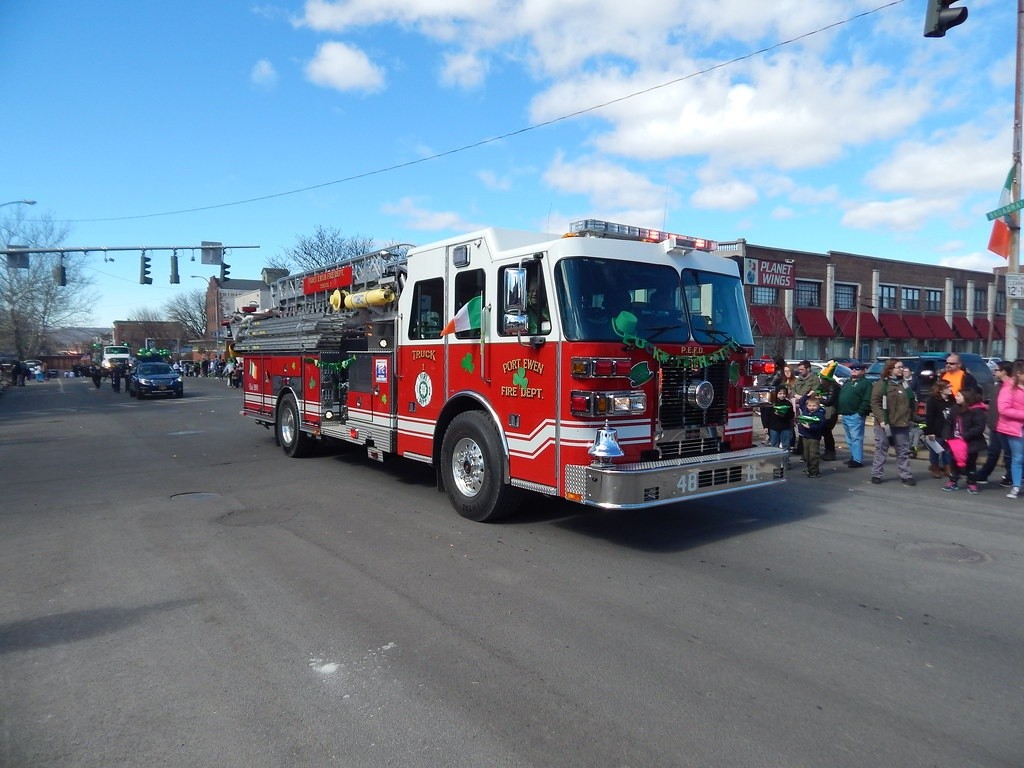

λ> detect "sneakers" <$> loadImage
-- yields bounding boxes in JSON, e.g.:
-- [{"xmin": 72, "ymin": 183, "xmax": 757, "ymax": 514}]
[
  {"xmin": 1006, "ymin": 486, "xmax": 1023, "ymax": 499},
  {"xmin": 963, "ymin": 475, "xmax": 989, "ymax": 484},
  {"xmin": 966, "ymin": 485, "xmax": 979, "ymax": 495},
  {"xmin": 941, "ymin": 480, "xmax": 959, "ymax": 492},
  {"xmin": 999, "ymin": 478, "xmax": 1012, "ymax": 488}
]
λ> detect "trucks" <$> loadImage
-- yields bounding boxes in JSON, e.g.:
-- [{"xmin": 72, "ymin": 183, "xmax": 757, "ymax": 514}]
[{"xmin": 101, "ymin": 345, "xmax": 130, "ymax": 370}]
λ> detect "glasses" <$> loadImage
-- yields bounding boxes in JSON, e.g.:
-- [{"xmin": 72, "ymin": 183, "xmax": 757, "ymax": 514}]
[
  {"xmin": 945, "ymin": 361, "xmax": 958, "ymax": 365},
  {"xmin": 993, "ymin": 369, "xmax": 1003, "ymax": 371},
  {"xmin": 944, "ymin": 385, "xmax": 953, "ymax": 389},
  {"xmin": 893, "ymin": 366, "xmax": 903, "ymax": 369},
  {"xmin": 850, "ymin": 367, "xmax": 863, "ymax": 370},
  {"xmin": 528, "ymin": 289, "xmax": 537, "ymax": 294}
]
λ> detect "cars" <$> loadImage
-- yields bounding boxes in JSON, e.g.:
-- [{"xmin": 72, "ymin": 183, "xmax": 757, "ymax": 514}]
[
  {"xmin": 127, "ymin": 362, "xmax": 183, "ymax": 400},
  {"xmin": 831, "ymin": 358, "xmax": 855, "ymax": 370},
  {"xmin": 24, "ymin": 360, "xmax": 41, "ymax": 365},
  {"xmin": 784, "ymin": 362, "xmax": 853, "ymax": 387},
  {"xmin": 982, "ymin": 357, "xmax": 1002, "ymax": 373},
  {"xmin": 50, "ymin": 370, "xmax": 57, "ymax": 375},
  {"xmin": 847, "ymin": 359, "xmax": 868, "ymax": 370},
  {"xmin": 864, "ymin": 356, "xmax": 948, "ymax": 403}
]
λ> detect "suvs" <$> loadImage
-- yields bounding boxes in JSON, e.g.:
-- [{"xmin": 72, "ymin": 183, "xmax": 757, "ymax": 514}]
[{"xmin": 124, "ymin": 353, "xmax": 173, "ymax": 389}]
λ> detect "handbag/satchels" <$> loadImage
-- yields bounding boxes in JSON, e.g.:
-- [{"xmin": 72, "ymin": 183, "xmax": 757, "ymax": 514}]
[{"xmin": 945, "ymin": 438, "xmax": 968, "ymax": 468}]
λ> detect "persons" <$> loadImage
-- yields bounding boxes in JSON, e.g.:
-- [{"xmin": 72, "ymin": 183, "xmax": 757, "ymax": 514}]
[
  {"xmin": 173, "ymin": 356, "xmax": 243, "ymax": 389},
  {"xmin": 63, "ymin": 370, "xmax": 75, "ymax": 378},
  {"xmin": 35, "ymin": 364, "xmax": 43, "ymax": 382},
  {"xmin": 527, "ymin": 276, "xmax": 550, "ymax": 333},
  {"xmin": 760, "ymin": 356, "xmax": 1024, "ymax": 499},
  {"xmin": 92, "ymin": 362, "xmax": 132, "ymax": 394},
  {"xmin": 10, "ymin": 359, "xmax": 27, "ymax": 386}
]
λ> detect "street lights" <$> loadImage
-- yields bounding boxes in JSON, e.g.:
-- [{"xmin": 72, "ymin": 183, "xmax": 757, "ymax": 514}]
[{"xmin": 191, "ymin": 276, "xmax": 219, "ymax": 358}]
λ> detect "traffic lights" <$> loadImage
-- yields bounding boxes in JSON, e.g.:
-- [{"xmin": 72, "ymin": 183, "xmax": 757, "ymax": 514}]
[
  {"xmin": 220, "ymin": 262, "xmax": 230, "ymax": 282},
  {"xmin": 56, "ymin": 265, "xmax": 67, "ymax": 286},
  {"xmin": 923, "ymin": 0, "xmax": 968, "ymax": 38},
  {"xmin": 141, "ymin": 256, "xmax": 152, "ymax": 285}
]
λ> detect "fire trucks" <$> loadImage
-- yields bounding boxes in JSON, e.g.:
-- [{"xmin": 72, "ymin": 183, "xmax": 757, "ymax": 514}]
[{"xmin": 233, "ymin": 219, "xmax": 790, "ymax": 521}]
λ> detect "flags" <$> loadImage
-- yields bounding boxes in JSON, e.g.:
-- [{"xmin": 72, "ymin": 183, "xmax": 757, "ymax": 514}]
[
  {"xmin": 440, "ymin": 296, "xmax": 481, "ymax": 336},
  {"xmin": 987, "ymin": 166, "xmax": 1015, "ymax": 261}
]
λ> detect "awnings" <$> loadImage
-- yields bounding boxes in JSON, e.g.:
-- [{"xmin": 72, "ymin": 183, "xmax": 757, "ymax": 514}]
[
  {"xmin": 751, "ymin": 306, "xmax": 794, "ymax": 337},
  {"xmin": 834, "ymin": 310, "xmax": 1006, "ymax": 340},
  {"xmin": 793, "ymin": 308, "xmax": 836, "ymax": 337}
]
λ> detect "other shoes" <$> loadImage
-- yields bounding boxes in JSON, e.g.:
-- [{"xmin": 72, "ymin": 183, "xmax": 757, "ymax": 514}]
[
  {"xmin": 808, "ymin": 473, "xmax": 821, "ymax": 478},
  {"xmin": 900, "ymin": 477, "xmax": 916, "ymax": 486},
  {"xmin": 768, "ymin": 440, "xmax": 805, "ymax": 469},
  {"xmin": 843, "ymin": 458, "xmax": 863, "ymax": 468},
  {"xmin": 928, "ymin": 464, "xmax": 945, "ymax": 478},
  {"xmin": 909, "ymin": 446, "xmax": 918, "ymax": 459},
  {"xmin": 820, "ymin": 448, "xmax": 836, "ymax": 461},
  {"xmin": 802, "ymin": 470, "xmax": 810, "ymax": 475},
  {"xmin": 871, "ymin": 477, "xmax": 880, "ymax": 484}
]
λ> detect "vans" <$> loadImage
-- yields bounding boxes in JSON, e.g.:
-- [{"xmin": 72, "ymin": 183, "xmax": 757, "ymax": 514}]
[
  {"xmin": 179, "ymin": 360, "xmax": 194, "ymax": 372},
  {"xmin": 910, "ymin": 352, "xmax": 994, "ymax": 405}
]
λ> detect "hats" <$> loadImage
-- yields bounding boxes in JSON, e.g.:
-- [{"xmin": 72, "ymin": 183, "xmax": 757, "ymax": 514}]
[
  {"xmin": 772, "ymin": 404, "xmax": 791, "ymax": 414},
  {"xmin": 849, "ymin": 362, "xmax": 868, "ymax": 369},
  {"xmin": 796, "ymin": 414, "xmax": 821, "ymax": 426},
  {"xmin": 611, "ymin": 311, "xmax": 638, "ymax": 339},
  {"xmin": 816, "ymin": 359, "xmax": 839, "ymax": 382}
]
